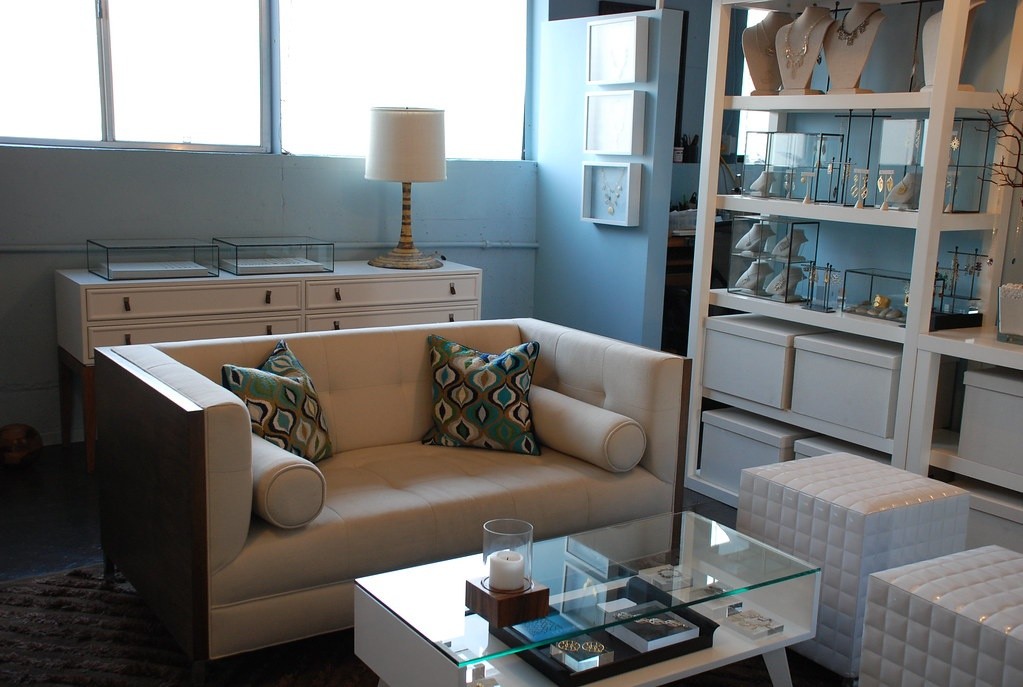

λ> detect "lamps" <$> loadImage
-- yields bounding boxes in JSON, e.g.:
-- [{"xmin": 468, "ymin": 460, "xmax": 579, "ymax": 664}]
[{"xmin": 364, "ymin": 106, "xmax": 447, "ymax": 270}]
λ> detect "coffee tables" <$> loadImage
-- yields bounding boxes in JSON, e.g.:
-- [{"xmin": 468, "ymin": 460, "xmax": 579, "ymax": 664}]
[{"xmin": 354, "ymin": 514, "xmax": 823, "ymax": 687}]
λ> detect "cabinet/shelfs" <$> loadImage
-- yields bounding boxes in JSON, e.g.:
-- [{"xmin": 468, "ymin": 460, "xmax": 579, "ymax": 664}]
[
  {"xmin": 726, "ymin": 213, "xmax": 820, "ymax": 304},
  {"xmin": 53, "ymin": 256, "xmax": 482, "ymax": 473},
  {"xmin": 685, "ymin": 0, "xmax": 1022, "ymax": 510}
]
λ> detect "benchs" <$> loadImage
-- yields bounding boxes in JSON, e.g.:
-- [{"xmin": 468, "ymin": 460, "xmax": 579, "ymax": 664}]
[{"xmin": 94, "ymin": 316, "xmax": 693, "ymax": 687}]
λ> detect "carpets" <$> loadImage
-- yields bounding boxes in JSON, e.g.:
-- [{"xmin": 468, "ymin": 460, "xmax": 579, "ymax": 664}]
[{"xmin": 0, "ymin": 563, "xmax": 856, "ymax": 687}]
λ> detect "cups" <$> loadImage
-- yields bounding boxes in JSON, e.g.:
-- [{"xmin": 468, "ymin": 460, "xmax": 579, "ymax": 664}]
[
  {"xmin": 683, "ymin": 145, "xmax": 698, "ymax": 163},
  {"xmin": 674, "ymin": 147, "xmax": 684, "ymax": 162}
]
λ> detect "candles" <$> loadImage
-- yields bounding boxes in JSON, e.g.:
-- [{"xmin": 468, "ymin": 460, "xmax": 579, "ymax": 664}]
[{"xmin": 487, "ymin": 549, "xmax": 524, "ymax": 591}]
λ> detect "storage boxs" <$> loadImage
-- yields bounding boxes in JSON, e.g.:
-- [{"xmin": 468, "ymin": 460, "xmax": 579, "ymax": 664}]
[
  {"xmin": 874, "ymin": 117, "xmax": 991, "ymax": 213},
  {"xmin": 699, "ymin": 406, "xmax": 821, "ymax": 495},
  {"xmin": 946, "ymin": 478, "xmax": 1023, "ymax": 554},
  {"xmin": 549, "ymin": 597, "xmax": 699, "ymax": 672},
  {"xmin": 791, "ymin": 332, "xmax": 904, "ymax": 440},
  {"xmin": 86, "ymin": 237, "xmax": 220, "ymax": 281},
  {"xmin": 740, "ymin": 131, "xmax": 844, "ymax": 203},
  {"xmin": 793, "ymin": 436, "xmax": 892, "ymax": 466},
  {"xmin": 957, "ymin": 367, "xmax": 1023, "ymax": 476},
  {"xmin": 212, "ymin": 235, "xmax": 335, "ymax": 276},
  {"xmin": 701, "ymin": 313, "xmax": 834, "ymax": 411},
  {"xmin": 841, "ymin": 267, "xmax": 946, "ymax": 323}
]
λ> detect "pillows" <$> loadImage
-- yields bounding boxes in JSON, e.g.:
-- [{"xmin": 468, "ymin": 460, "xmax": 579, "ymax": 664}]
[
  {"xmin": 530, "ymin": 383, "xmax": 646, "ymax": 474},
  {"xmin": 221, "ymin": 340, "xmax": 334, "ymax": 463},
  {"xmin": 251, "ymin": 433, "xmax": 326, "ymax": 530},
  {"xmin": 422, "ymin": 333, "xmax": 542, "ymax": 457}
]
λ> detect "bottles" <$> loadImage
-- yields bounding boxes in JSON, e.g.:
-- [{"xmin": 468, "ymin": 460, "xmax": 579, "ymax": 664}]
[{"xmin": 732, "ymin": 173, "xmax": 743, "ymax": 194}]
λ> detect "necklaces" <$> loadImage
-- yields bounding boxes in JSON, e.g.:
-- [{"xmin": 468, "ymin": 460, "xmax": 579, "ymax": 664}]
[
  {"xmin": 837, "ymin": 9, "xmax": 882, "ymax": 46},
  {"xmin": 760, "ymin": 20, "xmax": 775, "ymax": 55},
  {"xmin": 756, "ymin": 23, "xmax": 777, "ymax": 85},
  {"xmin": 779, "ymin": 236, "xmax": 789, "ymax": 251},
  {"xmin": 775, "ymin": 273, "xmax": 799, "ymax": 291},
  {"xmin": 741, "ymin": 270, "xmax": 760, "ymax": 285},
  {"xmin": 785, "ymin": 13, "xmax": 830, "ymax": 79},
  {"xmin": 745, "ymin": 232, "xmax": 759, "ymax": 246},
  {"xmin": 897, "ymin": 180, "xmax": 913, "ymax": 195}
]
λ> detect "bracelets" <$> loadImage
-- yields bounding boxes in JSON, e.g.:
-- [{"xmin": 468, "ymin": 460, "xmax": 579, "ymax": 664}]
[{"xmin": 658, "ymin": 569, "xmax": 682, "ymax": 578}]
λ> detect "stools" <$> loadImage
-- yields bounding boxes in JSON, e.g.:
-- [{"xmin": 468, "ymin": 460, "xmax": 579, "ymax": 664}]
[
  {"xmin": 735, "ymin": 451, "xmax": 971, "ymax": 679},
  {"xmin": 859, "ymin": 544, "xmax": 1023, "ymax": 687}
]
